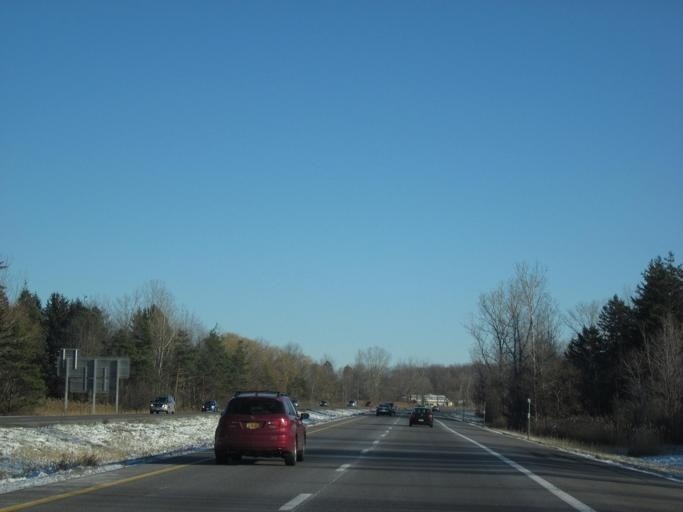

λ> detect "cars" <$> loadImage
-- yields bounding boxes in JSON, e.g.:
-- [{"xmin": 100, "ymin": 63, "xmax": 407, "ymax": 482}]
[
  {"xmin": 202, "ymin": 400, "xmax": 217, "ymax": 412},
  {"xmin": 375, "ymin": 404, "xmax": 392, "ymax": 416}
]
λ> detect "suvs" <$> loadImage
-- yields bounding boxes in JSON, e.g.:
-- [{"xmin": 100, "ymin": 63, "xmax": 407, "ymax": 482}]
[
  {"xmin": 408, "ymin": 407, "xmax": 434, "ymax": 428},
  {"xmin": 150, "ymin": 395, "xmax": 176, "ymax": 414},
  {"xmin": 214, "ymin": 389, "xmax": 310, "ymax": 466}
]
